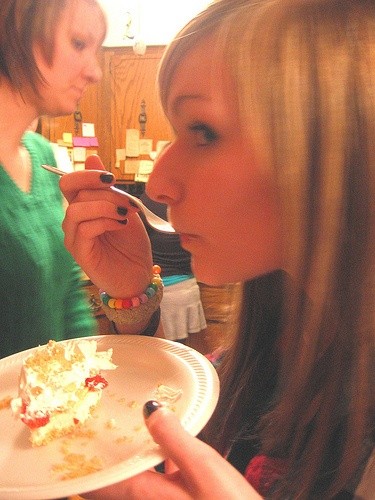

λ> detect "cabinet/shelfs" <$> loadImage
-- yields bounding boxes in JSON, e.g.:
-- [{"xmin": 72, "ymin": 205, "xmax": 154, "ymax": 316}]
[{"xmin": 41, "ymin": 45, "xmax": 176, "ymax": 185}]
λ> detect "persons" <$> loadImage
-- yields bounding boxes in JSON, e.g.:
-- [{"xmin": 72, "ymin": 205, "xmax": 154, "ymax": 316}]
[
  {"xmin": 0, "ymin": 0, "xmax": 108, "ymax": 358},
  {"xmin": 138, "ymin": 192, "xmax": 208, "ymax": 356},
  {"xmin": 60, "ymin": 0, "xmax": 375, "ymax": 500}
]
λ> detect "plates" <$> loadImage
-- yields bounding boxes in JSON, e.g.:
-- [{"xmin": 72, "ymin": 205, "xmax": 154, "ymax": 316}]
[{"xmin": 0, "ymin": 335, "xmax": 220, "ymax": 500}]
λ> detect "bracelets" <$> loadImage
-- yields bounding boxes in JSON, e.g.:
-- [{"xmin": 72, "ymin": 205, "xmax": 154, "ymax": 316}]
[{"xmin": 98, "ymin": 265, "xmax": 166, "ymax": 335}]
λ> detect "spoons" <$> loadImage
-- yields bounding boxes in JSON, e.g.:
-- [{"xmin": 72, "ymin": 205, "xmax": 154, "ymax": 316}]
[{"xmin": 41, "ymin": 164, "xmax": 178, "ymax": 235}]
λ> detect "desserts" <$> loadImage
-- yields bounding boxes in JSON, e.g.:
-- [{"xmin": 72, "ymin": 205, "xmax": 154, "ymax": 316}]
[{"xmin": 9, "ymin": 337, "xmax": 116, "ymax": 448}]
